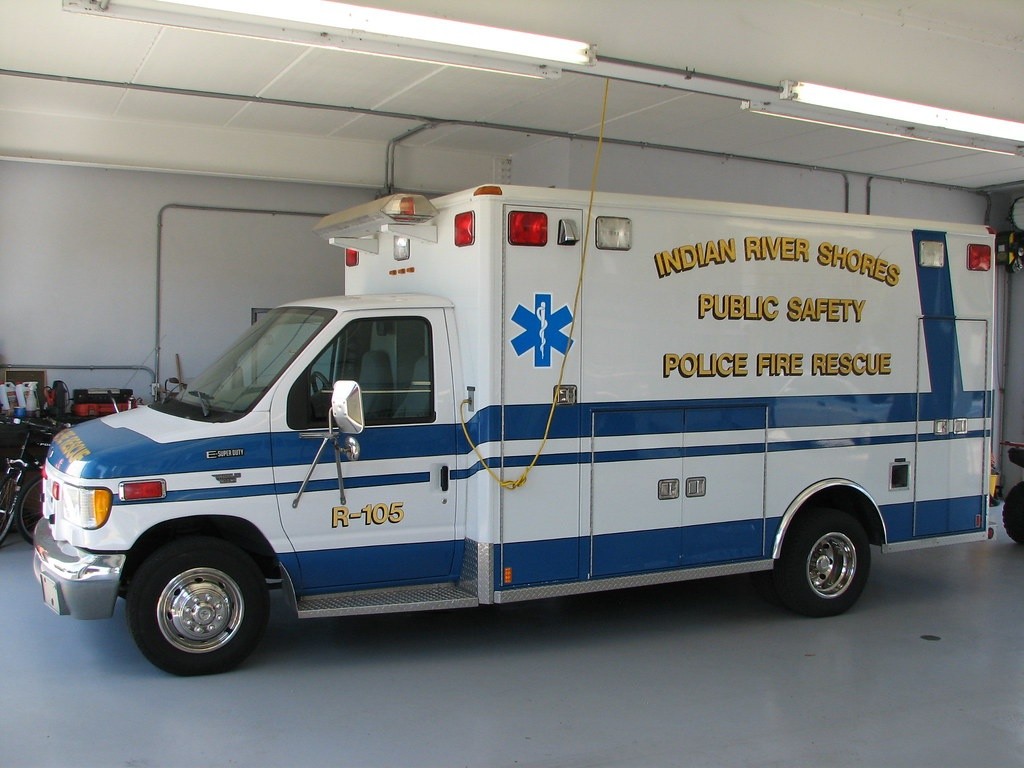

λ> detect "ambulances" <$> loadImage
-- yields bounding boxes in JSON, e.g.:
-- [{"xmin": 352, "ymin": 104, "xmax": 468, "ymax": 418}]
[{"xmin": 33, "ymin": 184, "xmax": 997, "ymax": 676}]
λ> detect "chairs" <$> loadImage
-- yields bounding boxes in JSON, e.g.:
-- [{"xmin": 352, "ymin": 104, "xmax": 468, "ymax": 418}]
[
  {"xmin": 395, "ymin": 355, "xmax": 433, "ymax": 420},
  {"xmin": 357, "ymin": 350, "xmax": 393, "ymax": 425}
]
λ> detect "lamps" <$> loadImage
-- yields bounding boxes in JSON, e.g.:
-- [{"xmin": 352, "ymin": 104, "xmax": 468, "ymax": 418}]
[
  {"xmin": 62, "ymin": 1, "xmax": 600, "ymax": 83},
  {"xmin": 737, "ymin": 79, "xmax": 1024, "ymax": 159}
]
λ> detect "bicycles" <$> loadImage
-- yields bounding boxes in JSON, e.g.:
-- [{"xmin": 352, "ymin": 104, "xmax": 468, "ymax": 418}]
[{"xmin": 0, "ymin": 408, "xmax": 97, "ymax": 546}]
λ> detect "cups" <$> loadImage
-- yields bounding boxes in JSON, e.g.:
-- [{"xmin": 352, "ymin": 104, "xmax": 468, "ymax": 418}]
[{"xmin": 13, "ymin": 406, "xmax": 27, "ymax": 418}]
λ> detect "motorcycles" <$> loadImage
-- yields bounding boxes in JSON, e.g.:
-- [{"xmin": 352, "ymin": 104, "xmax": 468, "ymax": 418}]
[{"xmin": 1000, "ymin": 440, "xmax": 1024, "ymax": 542}]
